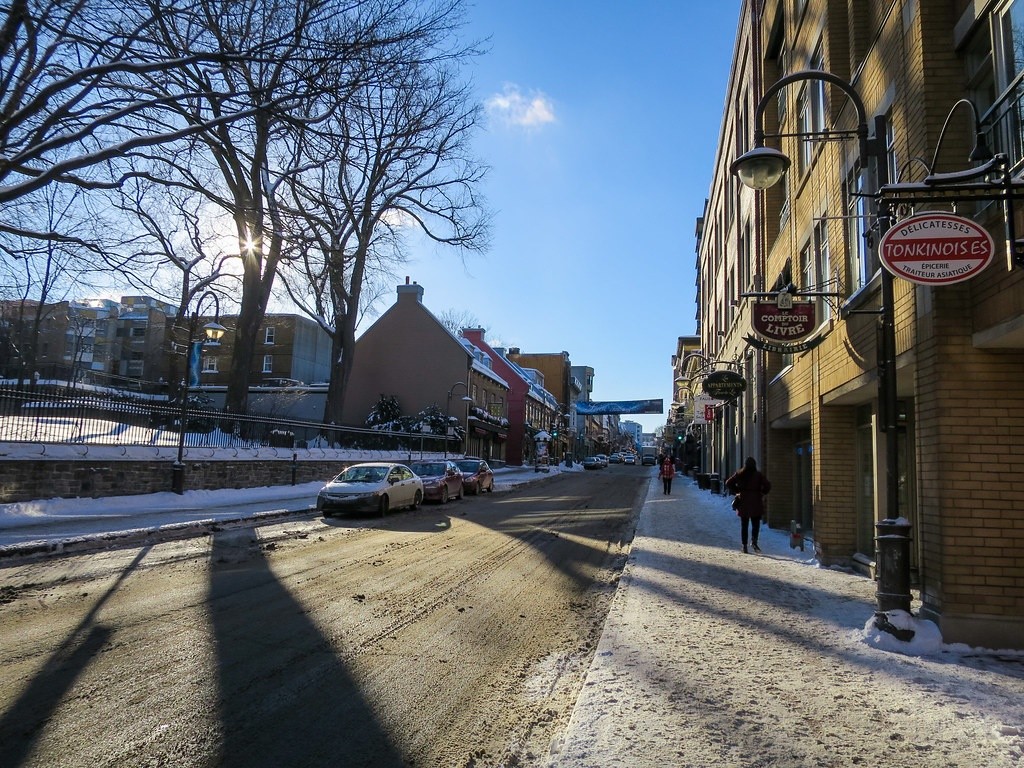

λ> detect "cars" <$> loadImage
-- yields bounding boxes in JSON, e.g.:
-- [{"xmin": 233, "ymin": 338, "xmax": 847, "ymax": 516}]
[
  {"xmin": 584, "ymin": 452, "xmax": 635, "ymax": 469},
  {"xmin": 317, "ymin": 462, "xmax": 424, "ymax": 518},
  {"xmin": 409, "ymin": 461, "xmax": 464, "ymax": 504},
  {"xmin": 455, "ymin": 460, "xmax": 494, "ymax": 494}
]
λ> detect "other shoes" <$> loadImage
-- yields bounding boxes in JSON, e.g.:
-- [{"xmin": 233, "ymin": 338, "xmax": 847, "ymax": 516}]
[
  {"xmin": 668, "ymin": 492, "xmax": 670, "ymax": 495},
  {"xmin": 663, "ymin": 492, "xmax": 666, "ymax": 495},
  {"xmin": 752, "ymin": 541, "xmax": 761, "ymax": 552},
  {"xmin": 744, "ymin": 546, "xmax": 748, "ymax": 553}
]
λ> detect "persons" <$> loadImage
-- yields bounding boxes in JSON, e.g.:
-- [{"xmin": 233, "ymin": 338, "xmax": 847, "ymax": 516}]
[
  {"xmin": 657, "ymin": 455, "xmax": 674, "ymax": 495},
  {"xmin": 725, "ymin": 457, "xmax": 771, "ymax": 554},
  {"xmin": 637, "ymin": 455, "xmax": 640, "ymax": 465}
]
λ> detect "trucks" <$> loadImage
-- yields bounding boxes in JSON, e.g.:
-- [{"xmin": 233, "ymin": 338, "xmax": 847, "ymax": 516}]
[{"xmin": 641, "ymin": 446, "xmax": 657, "ymax": 466}]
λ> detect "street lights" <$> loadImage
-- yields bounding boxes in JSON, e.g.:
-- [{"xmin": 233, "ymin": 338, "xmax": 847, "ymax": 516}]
[
  {"xmin": 172, "ymin": 291, "xmax": 228, "ymax": 495},
  {"xmin": 671, "ymin": 358, "xmax": 743, "ymax": 493},
  {"xmin": 445, "ymin": 382, "xmax": 472, "ymax": 458},
  {"xmin": 729, "ymin": 71, "xmax": 915, "ymax": 642}
]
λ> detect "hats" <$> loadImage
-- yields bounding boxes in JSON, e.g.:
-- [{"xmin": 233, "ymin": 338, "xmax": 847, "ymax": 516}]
[{"xmin": 664, "ymin": 458, "xmax": 670, "ymax": 462}]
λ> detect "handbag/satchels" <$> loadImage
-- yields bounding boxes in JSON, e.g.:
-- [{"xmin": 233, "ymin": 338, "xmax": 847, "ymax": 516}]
[{"xmin": 732, "ymin": 496, "xmax": 739, "ymax": 511}]
